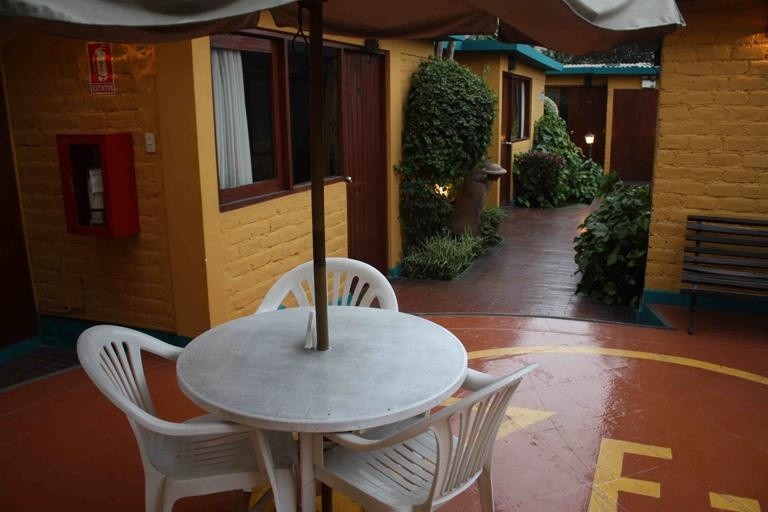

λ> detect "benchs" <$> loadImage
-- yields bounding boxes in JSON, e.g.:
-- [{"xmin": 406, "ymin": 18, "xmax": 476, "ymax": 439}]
[{"xmin": 680, "ymin": 212, "xmax": 768, "ymax": 334}]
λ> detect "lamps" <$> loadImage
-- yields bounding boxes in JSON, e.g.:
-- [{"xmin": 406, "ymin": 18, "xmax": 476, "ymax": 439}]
[{"xmin": 584, "ymin": 130, "xmax": 595, "ymax": 161}]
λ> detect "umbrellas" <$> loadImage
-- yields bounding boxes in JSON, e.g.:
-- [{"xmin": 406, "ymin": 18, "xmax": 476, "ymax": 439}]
[{"xmin": 0, "ymin": 1, "xmax": 684, "ymax": 351}]
